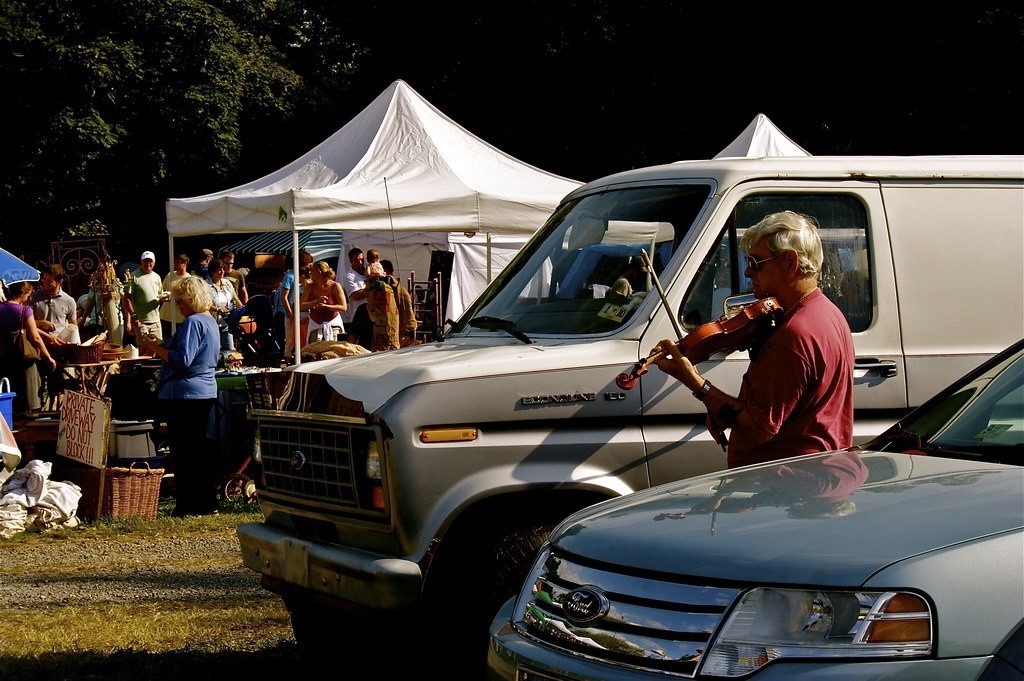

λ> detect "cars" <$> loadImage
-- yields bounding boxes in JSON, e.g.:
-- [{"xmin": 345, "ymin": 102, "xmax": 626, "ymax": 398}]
[{"xmin": 488, "ymin": 338, "xmax": 1023, "ymax": 681}]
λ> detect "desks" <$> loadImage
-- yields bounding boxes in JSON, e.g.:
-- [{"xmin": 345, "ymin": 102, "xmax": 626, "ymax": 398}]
[
  {"xmin": 12, "ymin": 418, "xmax": 60, "ymax": 466},
  {"xmin": 214, "ymin": 367, "xmax": 283, "ymax": 496},
  {"xmin": 53, "ymin": 355, "xmax": 153, "ymax": 413}
]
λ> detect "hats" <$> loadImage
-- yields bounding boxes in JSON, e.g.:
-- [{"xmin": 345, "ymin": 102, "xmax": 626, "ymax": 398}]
[{"xmin": 141, "ymin": 251, "xmax": 155, "ymax": 263}]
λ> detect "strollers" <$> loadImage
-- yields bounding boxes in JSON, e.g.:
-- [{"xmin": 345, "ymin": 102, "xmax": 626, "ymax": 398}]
[
  {"xmin": 229, "ymin": 295, "xmax": 283, "ymax": 366},
  {"xmin": 217, "ymin": 364, "xmax": 323, "ymax": 513}
]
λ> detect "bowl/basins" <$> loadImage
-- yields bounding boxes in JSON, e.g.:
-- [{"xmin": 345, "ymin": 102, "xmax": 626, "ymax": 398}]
[{"xmin": 103, "ymin": 343, "xmax": 123, "ymax": 352}]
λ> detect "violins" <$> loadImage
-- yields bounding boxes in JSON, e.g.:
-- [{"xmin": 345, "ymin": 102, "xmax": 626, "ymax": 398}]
[{"xmin": 615, "ymin": 295, "xmax": 786, "ymax": 391}]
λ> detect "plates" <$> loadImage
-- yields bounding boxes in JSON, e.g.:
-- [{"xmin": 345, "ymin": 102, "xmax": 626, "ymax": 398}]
[{"xmin": 102, "ymin": 351, "xmax": 129, "ymax": 360}]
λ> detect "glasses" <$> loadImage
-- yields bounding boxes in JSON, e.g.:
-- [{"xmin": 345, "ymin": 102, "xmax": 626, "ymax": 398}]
[
  {"xmin": 224, "ymin": 261, "xmax": 234, "ymax": 265},
  {"xmin": 744, "ymin": 255, "xmax": 776, "ymax": 272}
]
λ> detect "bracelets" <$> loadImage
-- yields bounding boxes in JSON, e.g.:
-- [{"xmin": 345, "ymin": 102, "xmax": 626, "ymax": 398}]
[
  {"xmin": 692, "ymin": 380, "xmax": 711, "ymax": 401},
  {"xmin": 131, "ymin": 313, "xmax": 136, "ymax": 317}
]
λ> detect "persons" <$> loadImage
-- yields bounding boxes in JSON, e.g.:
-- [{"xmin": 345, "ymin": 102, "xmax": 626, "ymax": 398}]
[
  {"xmin": 652, "ymin": 211, "xmax": 855, "ymax": 470},
  {"xmin": 246, "ymin": 282, "xmax": 285, "ymax": 350},
  {"xmin": 301, "ymin": 260, "xmax": 348, "ymax": 345},
  {"xmin": 78, "ymin": 262, "xmax": 132, "ymax": 346},
  {"xmin": 620, "ymin": 255, "xmax": 651, "ymax": 293},
  {"xmin": 365, "ymin": 250, "xmax": 386, "ymax": 276},
  {"xmin": 142, "ymin": 276, "xmax": 221, "ymax": 518},
  {"xmin": 124, "ymin": 251, "xmax": 171, "ymax": 348},
  {"xmin": 283, "ymin": 252, "xmax": 313, "ymax": 358},
  {"xmin": 30, "ymin": 264, "xmax": 78, "ymax": 400},
  {"xmin": 605, "ymin": 278, "xmax": 630, "ymax": 298},
  {"xmin": 346, "ymin": 248, "xmax": 418, "ymax": 351},
  {"xmin": 0, "ymin": 281, "xmax": 56, "ymax": 423},
  {"xmin": 159, "ymin": 249, "xmax": 249, "ymax": 338},
  {"xmin": 690, "ymin": 452, "xmax": 868, "ymax": 520}
]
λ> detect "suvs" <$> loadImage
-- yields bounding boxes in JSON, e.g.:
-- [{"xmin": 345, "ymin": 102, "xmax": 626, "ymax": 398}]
[{"xmin": 235, "ymin": 156, "xmax": 1024, "ymax": 680}]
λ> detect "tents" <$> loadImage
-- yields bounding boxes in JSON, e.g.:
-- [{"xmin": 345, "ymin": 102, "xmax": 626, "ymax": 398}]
[
  {"xmin": 711, "ymin": 113, "xmax": 813, "ymax": 158},
  {"xmin": 218, "ymin": 230, "xmax": 343, "ymax": 265},
  {"xmin": 336, "ymin": 229, "xmax": 560, "ymax": 333},
  {"xmin": 166, "ymin": 79, "xmax": 588, "ymax": 336}
]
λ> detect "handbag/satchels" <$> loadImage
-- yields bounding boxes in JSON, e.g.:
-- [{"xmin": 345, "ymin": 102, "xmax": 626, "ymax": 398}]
[{"xmin": 9, "ymin": 305, "xmax": 42, "ymax": 361}]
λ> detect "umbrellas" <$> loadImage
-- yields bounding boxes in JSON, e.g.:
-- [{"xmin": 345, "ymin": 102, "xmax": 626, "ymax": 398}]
[{"xmin": 0, "ymin": 247, "xmax": 41, "ymax": 286}]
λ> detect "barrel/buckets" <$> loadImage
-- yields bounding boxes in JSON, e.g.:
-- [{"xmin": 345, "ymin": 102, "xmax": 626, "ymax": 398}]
[{"xmin": 0, "ymin": 377, "xmax": 16, "ymax": 430}]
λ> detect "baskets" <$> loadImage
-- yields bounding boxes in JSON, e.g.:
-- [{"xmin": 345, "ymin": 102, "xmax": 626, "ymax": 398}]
[
  {"xmin": 77, "ymin": 461, "xmax": 165, "ymax": 524},
  {"xmin": 64, "ymin": 332, "xmax": 105, "ymax": 363}
]
